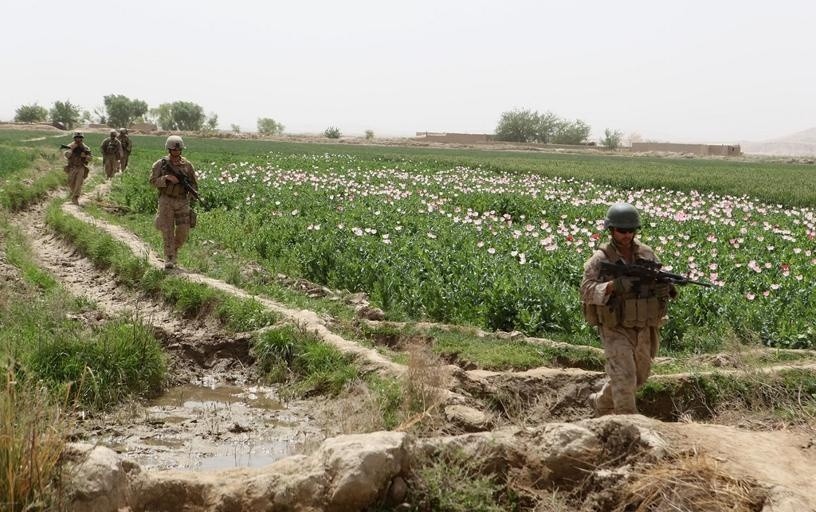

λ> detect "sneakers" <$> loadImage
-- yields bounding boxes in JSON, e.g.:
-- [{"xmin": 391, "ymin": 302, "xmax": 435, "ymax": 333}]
[
  {"xmin": 589, "ymin": 392, "xmax": 616, "ymax": 417},
  {"xmin": 72, "ymin": 196, "xmax": 79, "ymax": 206},
  {"xmin": 165, "ymin": 254, "xmax": 177, "ymax": 268}
]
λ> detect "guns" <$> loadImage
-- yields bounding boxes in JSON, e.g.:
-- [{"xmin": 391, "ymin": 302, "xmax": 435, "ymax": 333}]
[
  {"xmin": 161, "ymin": 164, "xmax": 210, "ymax": 212},
  {"xmin": 59, "ymin": 144, "xmax": 97, "ymax": 159},
  {"xmin": 597, "ymin": 258, "xmax": 710, "ymax": 313}
]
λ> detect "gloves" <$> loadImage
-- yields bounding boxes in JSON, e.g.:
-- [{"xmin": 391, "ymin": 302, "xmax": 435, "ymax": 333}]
[
  {"xmin": 613, "ymin": 276, "xmax": 649, "ymax": 297},
  {"xmin": 653, "ymin": 282, "xmax": 674, "ymax": 297}
]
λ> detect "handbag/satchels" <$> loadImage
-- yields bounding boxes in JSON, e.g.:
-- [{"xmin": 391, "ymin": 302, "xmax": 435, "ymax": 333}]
[
  {"xmin": 190, "ymin": 209, "xmax": 197, "ymax": 228},
  {"xmin": 84, "ymin": 167, "xmax": 89, "ymax": 179}
]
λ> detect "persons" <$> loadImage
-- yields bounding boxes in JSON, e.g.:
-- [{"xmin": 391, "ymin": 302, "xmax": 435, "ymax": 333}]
[
  {"xmin": 61, "ymin": 131, "xmax": 93, "ymax": 205},
  {"xmin": 99, "ymin": 130, "xmax": 123, "ymax": 181},
  {"xmin": 116, "ymin": 128, "xmax": 133, "ymax": 171},
  {"xmin": 577, "ymin": 201, "xmax": 679, "ymax": 416},
  {"xmin": 147, "ymin": 135, "xmax": 200, "ymax": 269}
]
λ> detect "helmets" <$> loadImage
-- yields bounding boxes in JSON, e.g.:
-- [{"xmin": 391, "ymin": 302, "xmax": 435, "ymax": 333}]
[
  {"xmin": 165, "ymin": 135, "xmax": 184, "ymax": 153},
  {"xmin": 110, "ymin": 127, "xmax": 128, "ymax": 136},
  {"xmin": 603, "ymin": 202, "xmax": 642, "ymax": 231},
  {"xmin": 72, "ymin": 131, "xmax": 84, "ymax": 139}
]
